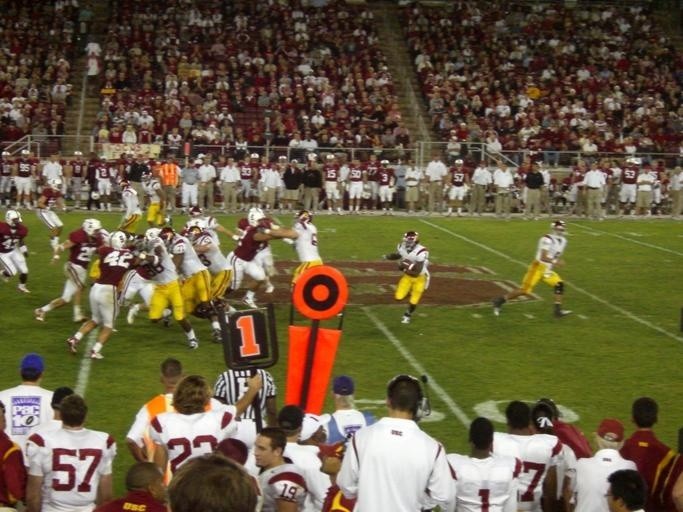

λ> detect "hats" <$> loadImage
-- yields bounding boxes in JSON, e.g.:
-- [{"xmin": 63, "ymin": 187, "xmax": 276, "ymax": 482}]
[
  {"xmin": 277, "ymin": 404, "xmax": 302, "ymax": 428},
  {"xmin": 598, "ymin": 419, "xmax": 624, "ymax": 442},
  {"xmin": 333, "ymin": 377, "xmax": 353, "ymax": 395},
  {"xmin": 21, "ymin": 354, "xmax": 43, "ymax": 371}
]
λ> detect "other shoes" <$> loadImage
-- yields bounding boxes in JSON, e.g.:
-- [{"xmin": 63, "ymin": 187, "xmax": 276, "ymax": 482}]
[{"xmin": 0, "ymin": 200, "xmax": 682, "ymax": 221}]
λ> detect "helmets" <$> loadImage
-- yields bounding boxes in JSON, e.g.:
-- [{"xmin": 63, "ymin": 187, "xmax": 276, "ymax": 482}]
[
  {"xmin": 140, "ymin": 171, "xmax": 152, "ymax": 182},
  {"xmin": 0, "ymin": 149, "xmax": 391, "ymax": 170},
  {"xmin": 403, "ymin": 231, "xmax": 418, "ymax": 244},
  {"xmin": 453, "ymin": 158, "xmax": 465, "ymax": 172},
  {"xmin": 551, "ymin": 219, "xmax": 566, "ymax": 232},
  {"xmin": 4, "ymin": 210, "xmax": 21, "ymax": 229},
  {"xmin": 294, "ymin": 209, "xmax": 312, "ymax": 223},
  {"xmin": 246, "ymin": 208, "xmax": 263, "ymax": 226},
  {"xmin": 623, "ymin": 156, "xmax": 683, "ymax": 176},
  {"xmin": 80, "ymin": 218, "xmax": 200, "ymax": 249},
  {"xmin": 118, "ymin": 178, "xmax": 130, "ymax": 191}
]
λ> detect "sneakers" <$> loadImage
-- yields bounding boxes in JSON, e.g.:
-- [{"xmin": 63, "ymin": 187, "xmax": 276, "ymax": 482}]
[
  {"xmin": 400, "ymin": 315, "xmax": 410, "ymax": 324},
  {"xmin": 492, "ymin": 298, "xmax": 501, "ymax": 316},
  {"xmin": 67, "ymin": 284, "xmax": 274, "ymax": 360},
  {"xmin": 555, "ymin": 310, "xmax": 572, "ymax": 317},
  {"xmin": 17, "ymin": 285, "xmax": 30, "ymax": 293},
  {"xmin": 34, "ymin": 307, "xmax": 46, "ymax": 321},
  {"xmin": 0, "ymin": 270, "xmax": 9, "ymax": 284}
]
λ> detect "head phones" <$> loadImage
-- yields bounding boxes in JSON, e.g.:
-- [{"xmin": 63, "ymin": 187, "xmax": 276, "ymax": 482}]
[{"xmin": 390, "ymin": 375, "xmax": 429, "ymax": 411}]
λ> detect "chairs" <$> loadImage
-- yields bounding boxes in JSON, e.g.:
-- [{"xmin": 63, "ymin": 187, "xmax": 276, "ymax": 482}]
[{"xmin": 0, "ymin": 0, "xmax": 682, "ymax": 166}]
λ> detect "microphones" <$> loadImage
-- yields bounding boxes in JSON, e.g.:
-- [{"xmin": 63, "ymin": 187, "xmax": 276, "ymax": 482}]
[{"xmin": 421, "ymin": 375, "xmax": 428, "ymax": 398}]
